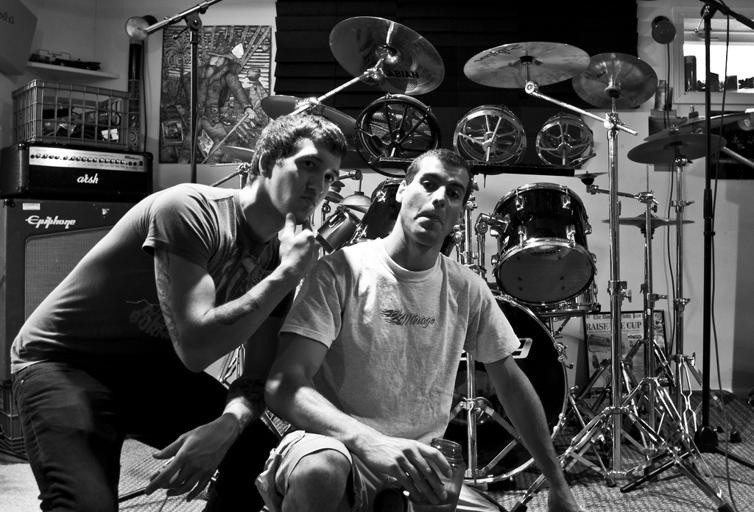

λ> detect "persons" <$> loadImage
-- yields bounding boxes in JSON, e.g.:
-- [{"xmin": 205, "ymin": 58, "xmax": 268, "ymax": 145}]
[
  {"xmin": 177, "ymin": 56, "xmax": 257, "ymax": 162},
  {"xmin": 256, "ymin": 147, "xmax": 585, "ymax": 512},
  {"xmin": 10, "ymin": 112, "xmax": 348, "ymax": 511}
]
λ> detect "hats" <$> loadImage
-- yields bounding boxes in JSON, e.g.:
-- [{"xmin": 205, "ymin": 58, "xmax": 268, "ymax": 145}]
[{"xmin": 207, "ymin": 44, "xmax": 234, "ymax": 61}]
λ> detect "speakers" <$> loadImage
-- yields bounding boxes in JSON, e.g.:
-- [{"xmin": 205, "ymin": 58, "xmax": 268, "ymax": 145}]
[{"xmin": 0, "ymin": 199, "xmax": 137, "ymax": 460}]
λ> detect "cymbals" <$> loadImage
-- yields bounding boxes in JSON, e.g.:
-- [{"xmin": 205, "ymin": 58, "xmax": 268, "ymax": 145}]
[
  {"xmin": 261, "ymin": 95, "xmax": 356, "ymax": 134},
  {"xmin": 464, "ymin": 42, "xmax": 590, "ymax": 89},
  {"xmin": 574, "ymin": 54, "xmax": 657, "ymax": 108},
  {"xmin": 330, "ymin": 18, "xmax": 443, "ymax": 97},
  {"xmin": 221, "ymin": 144, "xmax": 254, "ymax": 162},
  {"xmin": 628, "ymin": 111, "xmax": 751, "ymax": 165}
]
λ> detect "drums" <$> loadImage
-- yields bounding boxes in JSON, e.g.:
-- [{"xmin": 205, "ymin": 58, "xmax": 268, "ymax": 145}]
[
  {"xmin": 361, "ymin": 176, "xmax": 454, "ymax": 255},
  {"xmin": 493, "ymin": 184, "xmax": 595, "ymax": 305},
  {"xmin": 315, "ymin": 206, "xmax": 358, "ymax": 247},
  {"xmin": 443, "ymin": 295, "xmax": 569, "ymax": 487}
]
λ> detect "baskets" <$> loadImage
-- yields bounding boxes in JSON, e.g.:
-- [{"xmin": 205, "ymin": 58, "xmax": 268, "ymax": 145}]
[{"xmin": 8, "ymin": 78, "xmax": 133, "ymax": 152}]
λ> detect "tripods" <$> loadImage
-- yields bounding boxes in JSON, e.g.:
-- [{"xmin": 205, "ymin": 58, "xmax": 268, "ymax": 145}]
[
  {"xmin": 507, "ymin": 109, "xmax": 737, "ymax": 512},
  {"xmin": 477, "ymin": 9, "xmax": 754, "ymax": 494},
  {"xmin": 207, "ymin": 160, "xmax": 362, "ymax": 255},
  {"xmin": 115, "ymin": 32, "xmax": 274, "ymax": 512}
]
